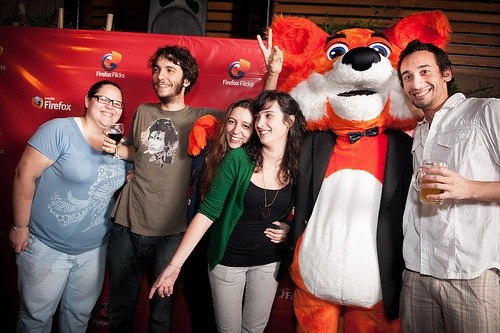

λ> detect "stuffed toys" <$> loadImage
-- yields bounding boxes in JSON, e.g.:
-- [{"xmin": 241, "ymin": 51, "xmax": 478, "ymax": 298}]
[{"xmin": 266, "ymin": 11, "xmax": 452, "ymax": 333}]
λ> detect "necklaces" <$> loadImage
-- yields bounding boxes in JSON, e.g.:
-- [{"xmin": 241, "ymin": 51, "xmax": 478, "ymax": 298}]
[{"xmin": 259, "ymin": 156, "xmax": 285, "ymax": 214}]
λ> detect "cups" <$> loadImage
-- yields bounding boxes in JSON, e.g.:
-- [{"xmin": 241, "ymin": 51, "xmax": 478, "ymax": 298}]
[{"xmin": 413, "ymin": 159, "xmax": 448, "ymax": 206}]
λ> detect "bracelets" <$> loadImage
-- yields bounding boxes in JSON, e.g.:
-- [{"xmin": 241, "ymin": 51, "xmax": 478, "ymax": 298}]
[
  {"xmin": 169, "ymin": 263, "xmax": 181, "ymax": 270},
  {"xmin": 11, "ymin": 224, "xmax": 28, "ymax": 230}
]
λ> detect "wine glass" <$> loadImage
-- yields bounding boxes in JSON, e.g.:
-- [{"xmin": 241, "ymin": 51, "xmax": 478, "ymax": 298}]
[{"xmin": 106, "ymin": 123, "xmax": 124, "ymax": 159}]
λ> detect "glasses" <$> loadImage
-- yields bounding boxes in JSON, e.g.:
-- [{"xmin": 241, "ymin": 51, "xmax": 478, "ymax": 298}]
[{"xmin": 92, "ymin": 95, "xmax": 125, "ymax": 109}]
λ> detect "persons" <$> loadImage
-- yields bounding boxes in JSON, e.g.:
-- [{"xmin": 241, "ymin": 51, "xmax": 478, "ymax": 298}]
[
  {"xmin": 149, "ymin": 90, "xmax": 307, "ymax": 333},
  {"xmin": 398, "ymin": 39, "xmax": 500, "ymax": 333},
  {"xmin": 102, "ymin": 30, "xmax": 283, "ymax": 333},
  {"xmin": 9, "ymin": 80, "xmax": 134, "ymax": 333},
  {"xmin": 127, "ymin": 99, "xmax": 290, "ymax": 243}
]
